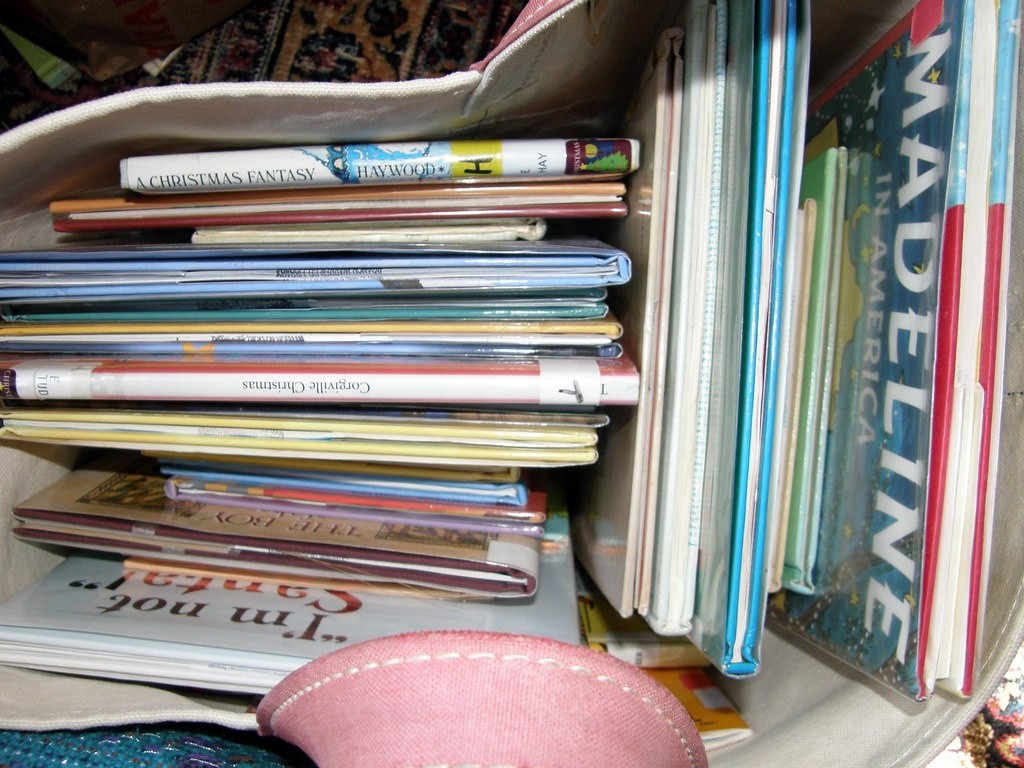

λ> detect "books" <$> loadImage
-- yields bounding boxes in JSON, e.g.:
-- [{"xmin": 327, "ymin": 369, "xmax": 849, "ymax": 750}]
[{"xmin": 0, "ymin": 0, "xmax": 1024, "ymax": 750}]
